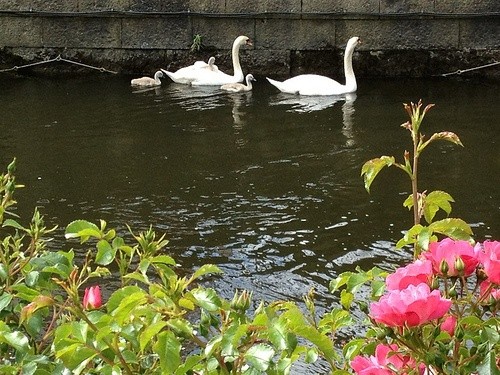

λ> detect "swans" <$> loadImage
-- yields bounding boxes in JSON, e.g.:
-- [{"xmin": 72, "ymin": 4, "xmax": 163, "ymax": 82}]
[
  {"xmin": 265, "ymin": 36, "xmax": 363, "ymax": 96},
  {"xmin": 129, "ymin": 34, "xmax": 257, "ymax": 94},
  {"xmin": 269, "ymin": 92, "xmax": 359, "ymax": 147}
]
